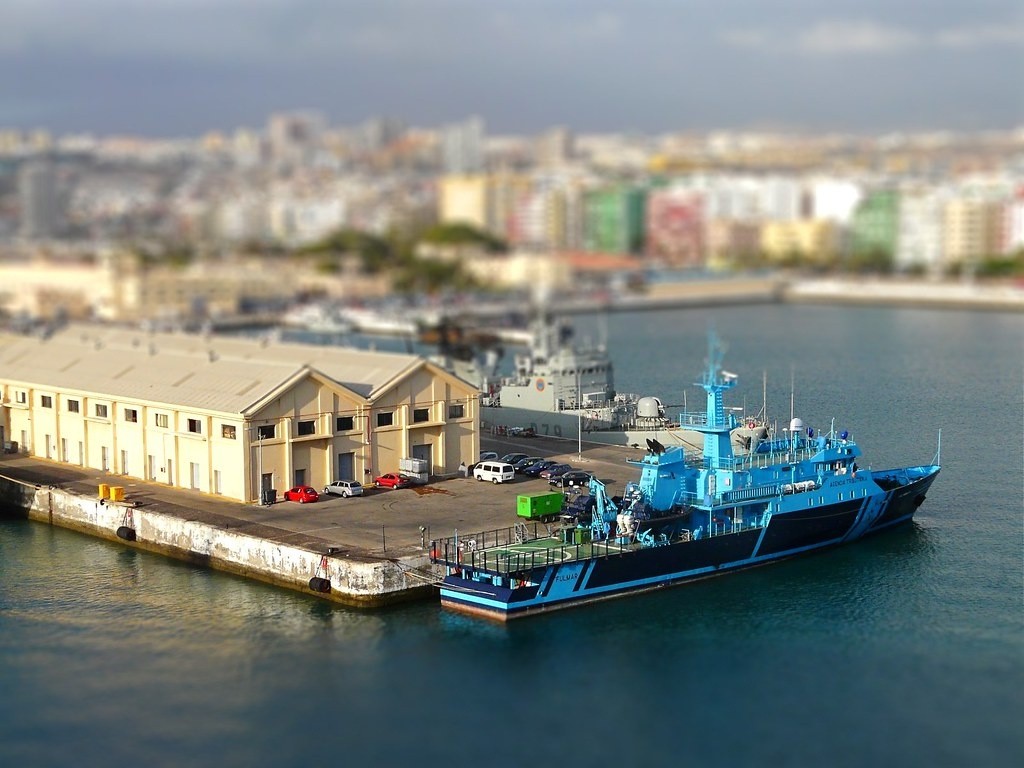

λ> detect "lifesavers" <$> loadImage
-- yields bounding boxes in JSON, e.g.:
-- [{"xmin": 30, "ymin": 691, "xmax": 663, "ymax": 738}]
[
  {"xmin": 309, "ymin": 577, "xmax": 320, "ymax": 591},
  {"xmin": 316, "ymin": 579, "xmax": 330, "ymax": 591},
  {"xmin": 749, "ymin": 423, "xmax": 755, "ymax": 428}
]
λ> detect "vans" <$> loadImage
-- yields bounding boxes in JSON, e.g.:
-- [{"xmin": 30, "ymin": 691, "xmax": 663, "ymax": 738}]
[{"xmin": 472, "ymin": 460, "xmax": 515, "ymax": 485}]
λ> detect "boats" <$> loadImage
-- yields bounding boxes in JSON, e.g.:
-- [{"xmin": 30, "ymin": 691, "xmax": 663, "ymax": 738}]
[
  {"xmin": 428, "ymin": 332, "xmax": 942, "ymax": 622},
  {"xmin": 479, "ymin": 296, "xmax": 770, "ymax": 458}
]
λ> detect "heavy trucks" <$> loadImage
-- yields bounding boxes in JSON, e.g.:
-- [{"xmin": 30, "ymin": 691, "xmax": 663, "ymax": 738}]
[{"xmin": 516, "ymin": 488, "xmax": 573, "ymax": 524}]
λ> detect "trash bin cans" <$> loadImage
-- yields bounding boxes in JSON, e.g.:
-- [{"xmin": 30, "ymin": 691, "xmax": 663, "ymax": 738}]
[
  {"xmin": 4, "ymin": 441, "xmax": 18, "ymax": 453},
  {"xmin": 264, "ymin": 489, "xmax": 278, "ymax": 504}
]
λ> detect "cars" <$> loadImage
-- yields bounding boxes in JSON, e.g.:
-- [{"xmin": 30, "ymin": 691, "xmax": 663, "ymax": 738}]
[
  {"xmin": 480, "ymin": 452, "xmax": 499, "ymax": 461},
  {"xmin": 283, "ymin": 486, "xmax": 319, "ymax": 503},
  {"xmin": 539, "ymin": 463, "xmax": 573, "ymax": 481},
  {"xmin": 496, "ymin": 453, "xmax": 530, "ymax": 465},
  {"xmin": 512, "ymin": 457, "xmax": 545, "ymax": 474},
  {"xmin": 548, "ymin": 470, "xmax": 597, "ymax": 487},
  {"xmin": 523, "ymin": 460, "xmax": 559, "ymax": 478}
]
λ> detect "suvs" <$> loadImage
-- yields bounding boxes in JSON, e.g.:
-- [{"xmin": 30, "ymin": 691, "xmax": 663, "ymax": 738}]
[
  {"xmin": 325, "ymin": 479, "xmax": 364, "ymax": 498},
  {"xmin": 374, "ymin": 473, "xmax": 411, "ymax": 490}
]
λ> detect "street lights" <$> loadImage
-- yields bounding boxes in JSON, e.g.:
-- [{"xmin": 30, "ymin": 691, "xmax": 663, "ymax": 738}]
[{"xmin": 257, "ymin": 428, "xmax": 267, "ymax": 506}]
